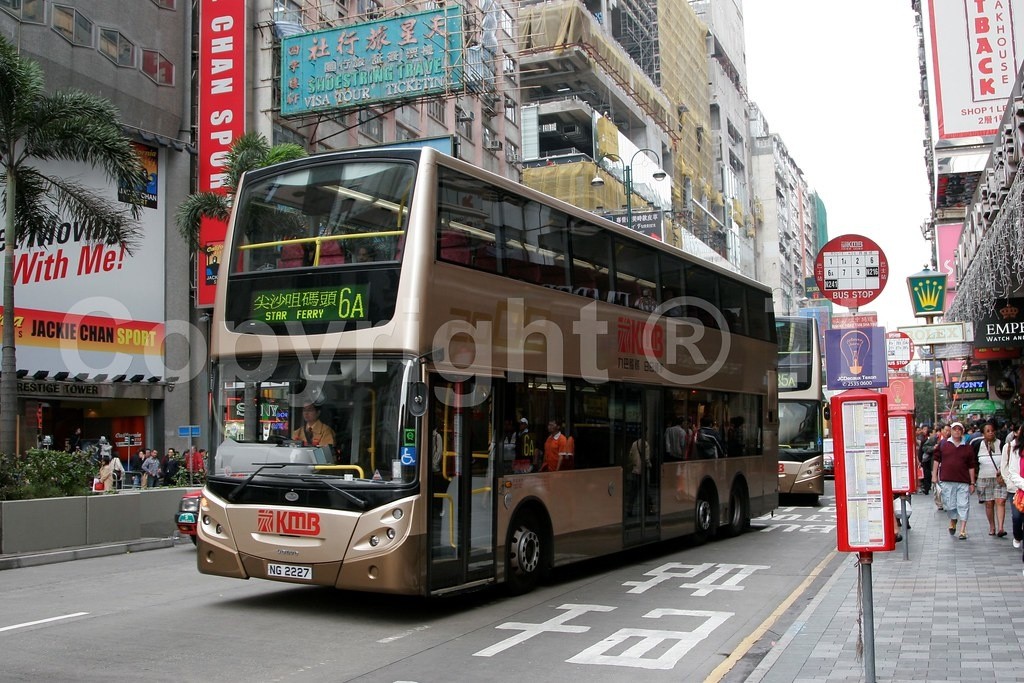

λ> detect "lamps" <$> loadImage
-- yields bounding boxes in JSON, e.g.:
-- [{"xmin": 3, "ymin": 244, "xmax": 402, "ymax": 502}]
[
  {"xmin": 16, "ymin": 368, "xmax": 29, "ymax": 380},
  {"xmin": 74, "ymin": 373, "xmax": 89, "ymax": 383},
  {"xmin": 185, "ymin": 144, "xmax": 198, "ymax": 156},
  {"xmin": 155, "ymin": 135, "xmax": 169, "ymax": 148},
  {"xmin": 130, "ymin": 374, "xmax": 145, "ymax": 383},
  {"xmin": 137, "ymin": 130, "xmax": 153, "ymax": 143},
  {"xmin": 166, "ymin": 376, "xmax": 180, "ymax": 383},
  {"xmin": 92, "ymin": 374, "xmax": 109, "ymax": 383},
  {"xmin": 54, "ymin": 371, "xmax": 71, "ymax": 382},
  {"xmin": 32, "ymin": 371, "xmax": 49, "ymax": 382},
  {"xmin": 112, "ymin": 374, "xmax": 127, "ymax": 383},
  {"xmin": 170, "ymin": 139, "xmax": 183, "ymax": 153},
  {"xmin": 148, "ymin": 376, "xmax": 162, "ymax": 383}
]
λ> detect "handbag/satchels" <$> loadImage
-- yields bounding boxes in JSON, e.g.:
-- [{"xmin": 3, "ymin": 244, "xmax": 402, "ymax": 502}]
[
  {"xmin": 1012, "ymin": 490, "xmax": 1024, "ymax": 512},
  {"xmin": 918, "ymin": 465, "xmax": 924, "ymax": 479},
  {"xmin": 112, "ymin": 457, "xmax": 122, "ymax": 481},
  {"xmin": 922, "ymin": 452, "xmax": 931, "ymax": 463},
  {"xmin": 92, "ymin": 478, "xmax": 104, "ymax": 492},
  {"xmin": 95, "ymin": 480, "xmax": 105, "ymax": 490},
  {"xmin": 996, "ymin": 470, "xmax": 1007, "ymax": 487}
]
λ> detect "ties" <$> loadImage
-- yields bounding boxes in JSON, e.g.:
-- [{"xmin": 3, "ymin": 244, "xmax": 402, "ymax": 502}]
[{"xmin": 308, "ymin": 427, "xmax": 312, "ymax": 439}]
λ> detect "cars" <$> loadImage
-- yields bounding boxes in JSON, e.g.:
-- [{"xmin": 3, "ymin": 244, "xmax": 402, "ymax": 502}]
[{"xmin": 175, "ymin": 489, "xmax": 205, "ymax": 546}]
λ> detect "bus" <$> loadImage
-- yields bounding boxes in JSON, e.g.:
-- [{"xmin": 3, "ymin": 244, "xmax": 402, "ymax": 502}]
[
  {"xmin": 820, "ymin": 352, "xmax": 834, "ymax": 478},
  {"xmin": 774, "ymin": 316, "xmax": 830, "ymax": 502},
  {"xmin": 196, "ymin": 146, "xmax": 780, "ymax": 598}
]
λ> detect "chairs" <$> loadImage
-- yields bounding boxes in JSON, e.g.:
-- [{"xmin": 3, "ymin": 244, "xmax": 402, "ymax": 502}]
[
  {"xmin": 440, "ymin": 222, "xmax": 476, "ymax": 265},
  {"xmin": 688, "ymin": 297, "xmax": 715, "ymax": 327},
  {"xmin": 319, "ymin": 399, "xmax": 351, "ymax": 464},
  {"xmin": 508, "ymin": 241, "xmax": 546, "ymax": 284},
  {"xmin": 598, "ymin": 264, "xmax": 610, "ymax": 302},
  {"xmin": 618, "ymin": 272, "xmax": 642, "ymax": 307},
  {"xmin": 721, "ymin": 309, "xmax": 737, "ymax": 332},
  {"xmin": 472, "ymin": 226, "xmax": 497, "ymax": 271},
  {"xmin": 544, "ymin": 262, "xmax": 567, "ymax": 292},
  {"xmin": 319, "ymin": 235, "xmax": 353, "ymax": 265},
  {"xmin": 277, "ymin": 236, "xmax": 308, "ymax": 269},
  {"xmin": 574, "ymin": 257, "xmax": 597, "ymax": 295}
]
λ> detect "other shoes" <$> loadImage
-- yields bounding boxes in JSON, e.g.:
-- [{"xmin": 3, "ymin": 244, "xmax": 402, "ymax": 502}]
[
  {"xmin": 938, "ymin": 506, "xmax": 943, "ymax": 510},
  {"xmin": 649, "ymin": 505, "xmax": 656, "ymax": 514},
  {"xmin": 998, "ymin": 531, "xmax": 1007, "ymax": 537},
  {"xmin": 949, "ymin": 527, "xmax": 956, "ymax": 535},
  {"xmin": 921, "ymin": 486, "xmax": 928, "ymax": 495},
  {"xmin": 625, "ymin": 508, "xmax": 637, "ymax": 516},
  {"xmin": 989, "ymin": 528, "xmax": 995, "ymax": 535},
  {"xmin": 959, "ymin": 532, "xmax": 966, "ymax": 539},
  {"xmin": 1013, "ymin": 538, "xmax": 1020, "ymax": 548}
]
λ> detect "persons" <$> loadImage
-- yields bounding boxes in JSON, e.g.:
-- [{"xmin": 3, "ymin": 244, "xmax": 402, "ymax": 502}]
[
  {"xmin": 432, "ymin": 423, "xmax": 442, "ymax": 474},
  {"xmin": 914, "ymin": 418, "xmax": 1024, "ymax": 576},
  {"xmin": 628, "ymin": 416, "xmax": 746, "ymax": 516},
  {"xmin": 293, "ymin": 398, "xmax": 336, "ymax": 455},
  {"xmin": 894, "ymin": 492, "xmax": 911, "ymax": 536},
  {"xmin": 99, "ymin": 451, "xmax": 126, "ymax": 495},
  {"xmin": 132, "ymin": 447, "xmax": 208, "ymax": 489},
  {"xmin": 512, "ymin": 418, "xmax": 574, "ymax": 474}
]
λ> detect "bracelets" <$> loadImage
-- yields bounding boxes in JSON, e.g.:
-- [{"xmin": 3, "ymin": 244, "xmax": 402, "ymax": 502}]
[{"xmin": 971, "ymin": 483, "xmax": 975, "ymax": 485}]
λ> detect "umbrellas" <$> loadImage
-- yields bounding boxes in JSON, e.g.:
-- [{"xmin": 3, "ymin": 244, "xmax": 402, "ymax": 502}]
[{"xmin": 961, "ymin": 399, "xmax": 1007, "ymax": 415}]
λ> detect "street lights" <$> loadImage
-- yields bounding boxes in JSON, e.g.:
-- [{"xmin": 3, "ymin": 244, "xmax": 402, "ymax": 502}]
[{"xmin": 591, "ymin": 148, "xmax": 667, "ymax": 227}]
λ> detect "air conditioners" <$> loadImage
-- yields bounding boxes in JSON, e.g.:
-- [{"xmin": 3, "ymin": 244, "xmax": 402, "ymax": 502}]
[
  {"xmin": 490, "ymin": 93, "xmax": 501, "ymax": 102},
  {"xmin": 505, "ymin": 99, "xmax": 514, "ymax": 107},
  {"xmin": 509, "ymin": 69, "xmax": 516, "ymax": 77},
  {"xmin": 461, "ymin": 110, "xmax": 475, "ymax": 121},
  {"xmin": 490, "ymin": 140, "xmax": 503, "ymax": 151},
  {"xmin": 514, "ymin": 154, "xmax": 521, "ymax": 164}
]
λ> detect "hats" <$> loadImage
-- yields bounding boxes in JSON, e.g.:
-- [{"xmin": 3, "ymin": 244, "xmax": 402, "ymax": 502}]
[
  {"xmin": 951, "ymin": 422, "xmax": 964, "ymax": 430},
  {"xmin": 518, "ymin": 417, "xmax": 528, "ymax": 425}
]
